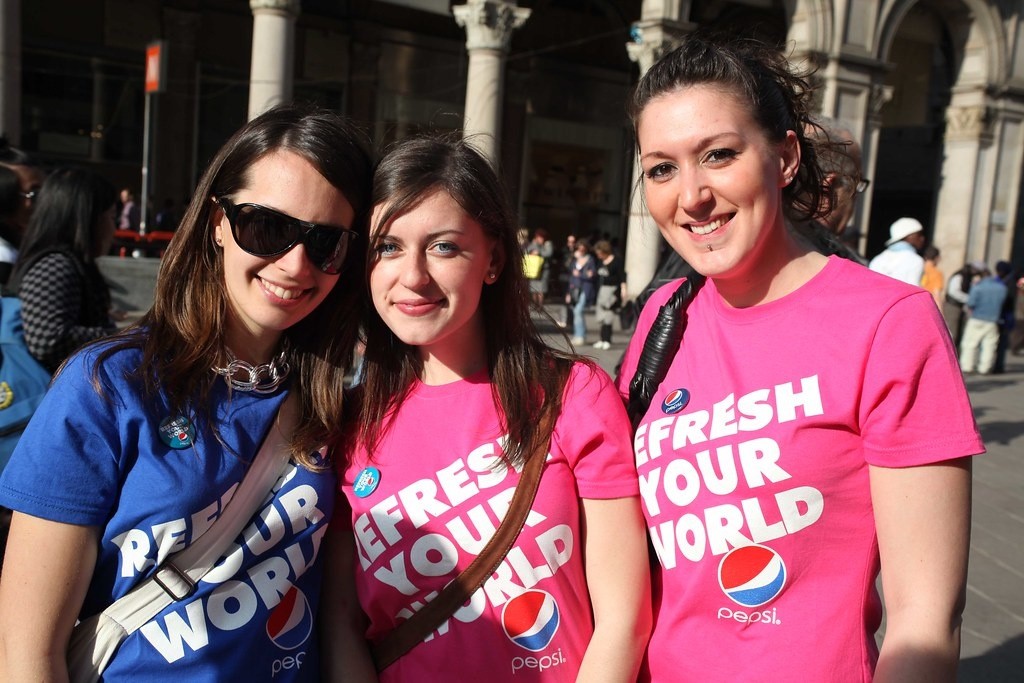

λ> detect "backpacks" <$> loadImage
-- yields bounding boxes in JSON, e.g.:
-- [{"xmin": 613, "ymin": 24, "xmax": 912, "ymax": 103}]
[{"xmin": 0, "ymin": 245, "xmax": 89, "ymax": 472}]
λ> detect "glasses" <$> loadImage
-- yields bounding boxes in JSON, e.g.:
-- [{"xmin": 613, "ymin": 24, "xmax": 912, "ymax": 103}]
[{"xmin": 210, "ymin": 197, "xmax": 359, "ymax": 273}]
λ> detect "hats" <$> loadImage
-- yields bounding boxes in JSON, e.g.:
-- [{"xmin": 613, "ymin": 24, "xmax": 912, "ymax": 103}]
[{"xmin": 886, "ymin": 217, "xmax": 922, "ymax": 245}]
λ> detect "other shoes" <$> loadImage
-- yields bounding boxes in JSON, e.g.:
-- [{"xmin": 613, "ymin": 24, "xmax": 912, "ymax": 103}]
[
  {"xmin": 531, "ymin": 305, "xmax": 544, "ymax": 313},
  {"xmin": 593, "ymin": 341, "xmax": 603, "ymax": 349},
  {"xmin": 603, "ymin": 341, "xmax": 610, "ymax": 350},
  {"xmin": 571, "ymin": 336, "xmax": 586, "ymax": 346}
]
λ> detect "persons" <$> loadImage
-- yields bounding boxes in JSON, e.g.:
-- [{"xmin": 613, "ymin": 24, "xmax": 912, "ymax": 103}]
[
  {"xmin": 0, "ymin": 144, "xmax": 120, "ymax": 544},
  {"xmin": 947, "ymin": 260, "xmax": 1024, "ymax": 373},
  {"xmin": 869, "ymin": 217, "xmax": 926, "ymax": 287},
  {"xmin": 921, "ymin": 247, "xmax": 946, "ymax": 316},
  {"xmin": 845, "ymin": 226, "xmax": 860, "ymax": 252},
  {"xmin": 0, "ymin": 102, "xmax": 375, "ymax": 683},
  {"xmin": 519, "ymin": 227, "xmax": 620, "ymax": 348},
  {"xmin": 313, "ymin": 139, "xmax": 654, "ymax": 683},
  {"xmin": 613, "ymin": 41, "xmax": 987, "ymax": 683},
  {"xmin": 790, "ymin": 115, "xmax": 861, "ymax": 238},
  {"xmin": 119, "ymin": 190, "xmax": 182, "ymax": 253}
]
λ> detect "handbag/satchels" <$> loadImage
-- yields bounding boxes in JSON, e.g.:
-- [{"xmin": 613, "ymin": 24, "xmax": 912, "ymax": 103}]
[
  {"xmin": 570, "ymin": 288, "xmax": 580, "ymax": 306},
  {"xmin": 523, "ymin": 254, "xmax": 544, "ymax": 279}
]
